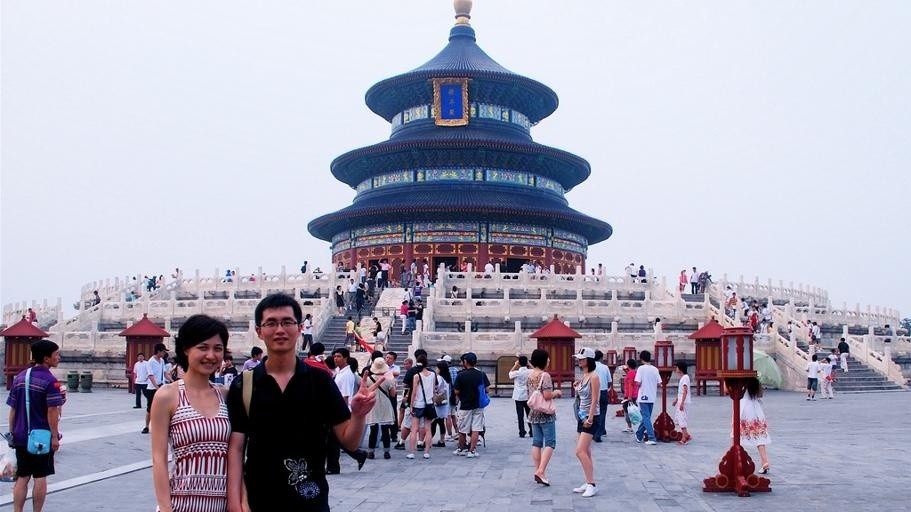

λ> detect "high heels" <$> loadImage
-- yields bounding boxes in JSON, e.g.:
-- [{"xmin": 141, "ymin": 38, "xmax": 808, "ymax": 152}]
[{"xmin": 759, "ymin": 463, "xmax": 769, "ymax": 475}]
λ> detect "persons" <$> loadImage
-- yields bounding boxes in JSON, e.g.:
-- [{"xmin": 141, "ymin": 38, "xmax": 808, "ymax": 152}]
[
  {"xmin": 723, "ymin": 266, "xmax": 850, "ymax": 401},
  {"xmin": 334, "ymin": 255, "xmax": 434, "ymax": 336},
  {"xmin": 572, "ymin": 347, "xmax": 691, "ymax": 497},
  {"xmin": 249, "ymin": 273, "xmax": 256, "ymax": 281},
  {"xmin": 652, "ymin": 316, "xmax": 666, "ymax": 333},
  {"xmin": 508, "ymin": 349, "xmax": 562, "ymax": 486},
  {"xmin": 5, "ymin": 340, "xmax": 66, "ymax": 512},
  {"xmin": 131, "ymin": 267, "xmax": 181, "ymax": 302},
  {"xmin": 227, "ymin": 293, "xmax": 385, "ymax": 512},
  {"xmin": 93, "ymin": 291, "xmax": 100, "ymax": 304},
  {"xmin": 149, "ymin": 313, "xmax": 252, "ymax": 512},
  {"xmin": 304, "ymin": 344, "xmax": 491, "ymax": 474},
  {"xmin": 355, "ymin": 322, "xmax": 363, "ymax": 352},
  {"xmin": 22, "ymin": 315, "xmax": 26, "ymax": 319},
  {"xmin": 224, "ymin": 270, "xmax": 232, "ymax": 282},
  {"xmin": 26, "ymin": 307, "xmax": 38, "ymax": 327},
  {"xmin": 209, "ymin": 346, "xmax": 263, "ymax": 390},
  {"xmin": 134, "ymin": 344, "xmax": 185, "ymax": 434},
  {"xmin": 723, "ymin": 372, "xmax": 772, "ymax": 474},
  {"xmin": 300, "ymin": 260, "xmax": 307, "ymax": 273},
  {"xmin": 445, "ymin": 259, "xmax": 714, "ymax": 298},
  {"xmin": 344, "ymin": 316, "xmax": 355, "ymax": 346},
  {"xmin": 302, "ymin": 313, "xmax": 314, "ymax": 350},
  {"xmin": 881, "ymin": 324, "xmax": 892, "ymax": 342},
  {"xmin": 232, "ymin": 271, "xmax": 236, "ymax": 276}
]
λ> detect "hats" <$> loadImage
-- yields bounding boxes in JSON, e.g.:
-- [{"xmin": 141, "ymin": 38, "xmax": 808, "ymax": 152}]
[
  {"xmin": 437, "ymin": 355, "xmax": 452, "ymax": 363},
  {"xmin": 369, "ymin": 357, "xmax": 388, "ymax": 374},
  {"xmin": 571, "ymin": 348, "xmax": 596, "ymax": 359},
  {"xmin": 154, "ymin": 343, "xmax": 170, "ymax": 352}
]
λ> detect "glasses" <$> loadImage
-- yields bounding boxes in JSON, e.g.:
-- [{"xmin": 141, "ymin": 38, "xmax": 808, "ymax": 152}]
[{"xmin": 258, "ymin": 320, "xmax": 299, "ymax": 327}]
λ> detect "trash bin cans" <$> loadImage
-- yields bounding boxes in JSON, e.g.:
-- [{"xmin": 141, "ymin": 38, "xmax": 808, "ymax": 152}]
[
  {"xmin": 79, "ymin": 370, "xmax": 93, "ymax": 393},
  {"xmin": 66, "ymin": 370, "xmax": 79, "ymax": 393}
]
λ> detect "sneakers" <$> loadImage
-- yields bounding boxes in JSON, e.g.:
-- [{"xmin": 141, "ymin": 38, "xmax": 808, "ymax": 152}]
[
  {"xmin": 582, "ymin": 484, "xmax": 598, "ymax": 497},
  {"xmin": 635, "ymin": 434, "xmax": 643, "ymax": 443},
  {"xmin": 368, "ymin": 452, "xmax": 374, "ymax": 459},
  {"xmin": 326, "ymin": 468, "xmax": 340, "ymax": 475},
  {"xmin": 645, "ymin": 439, "xmax": 656, "ymax": 445},
  {"xmin": 623, "ymin": 428, "xmax": 634, "ymax": 433},
  {"xmin": 358, "ymin": 451, "xmax": 367, "ymax": 470},
  {"xmin": 393, "ymin": 432, "xmax": 481, "ymax": 459},
  {"xmin": 384, "ymin": 452, "xmax": 391, "ymax": 459},
  {"xmin": 535, "ymin": 474, "xmax": 550, "ymax": 486},
  {"xmin": 806, "ymin": 396, "xmax": 832, "ymax": 401},
  {"xmin": 574, "ymin": 483, "xmax": 588, "ymax": 493},
  {"xmin": 141, "ymin": 427, "xmax": 149, "ymax": 433}
]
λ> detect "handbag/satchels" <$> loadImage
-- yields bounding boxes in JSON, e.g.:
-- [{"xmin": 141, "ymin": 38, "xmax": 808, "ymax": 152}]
[
  {"xmin": 527, "ymin": 390, "xmax": 556, "ymax": 415},
  {"xmin": 26, "ymin": 429, "xmax": 52, "ymax": 455},
  {"xmin": 423, "ymin": 403, "xmax": 438, "ymax": 418},
  {"xmin": 434, "ymin": 389, "xmax": 447, "ymax": 405},
  {"xmin": 607, "ymin": 387, "xmax": 618, "ymax": 403},
  {"xmin": 479, "ymin": 384, "xmax": 490, "ymax": 408}
]
